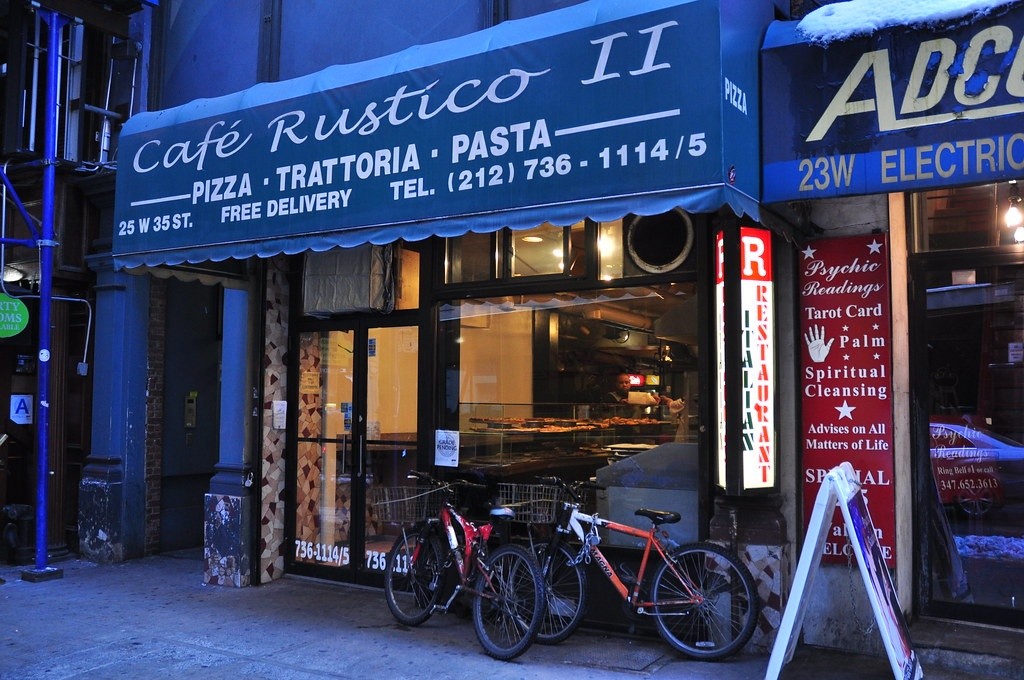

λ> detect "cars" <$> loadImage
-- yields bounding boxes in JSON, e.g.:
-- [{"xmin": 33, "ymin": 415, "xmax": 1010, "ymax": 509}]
[{"xmin": 926, "ymin": 421, "xmax": 1024, "ymax": 522}]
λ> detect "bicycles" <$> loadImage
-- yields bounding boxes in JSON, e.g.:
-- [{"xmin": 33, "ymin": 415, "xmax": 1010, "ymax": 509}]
[
  {"xmin": 373, "ymin": 469, "xmax": 548, "ymax": 662},
  {"xmin": 506, "ymin": 474, "xmax": 759, "ymax": 662}
]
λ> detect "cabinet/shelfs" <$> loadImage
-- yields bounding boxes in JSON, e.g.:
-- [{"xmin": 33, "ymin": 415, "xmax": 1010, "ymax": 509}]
[{"xmin": 450, "ymin": 421, "xmax": 672, "ymax": 477}]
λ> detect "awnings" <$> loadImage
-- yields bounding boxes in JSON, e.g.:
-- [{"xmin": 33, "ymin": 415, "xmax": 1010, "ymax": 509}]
[
  {"xmin": 105, "ymin": 0, "xmax": 809, "ymax": 292},
  {"xmin": 762, "ymin": 0, "xmax": 1024, "ymax": 242}
]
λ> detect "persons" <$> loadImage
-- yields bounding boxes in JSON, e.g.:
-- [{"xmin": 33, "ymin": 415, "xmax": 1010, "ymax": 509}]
[
  {"xmin": 597, "ymin": 372, "xmax": 631, "ymax": 404},
  {"xmin": 648, "ymin": 389, "xmax": 676, "ymax": 407}
]
